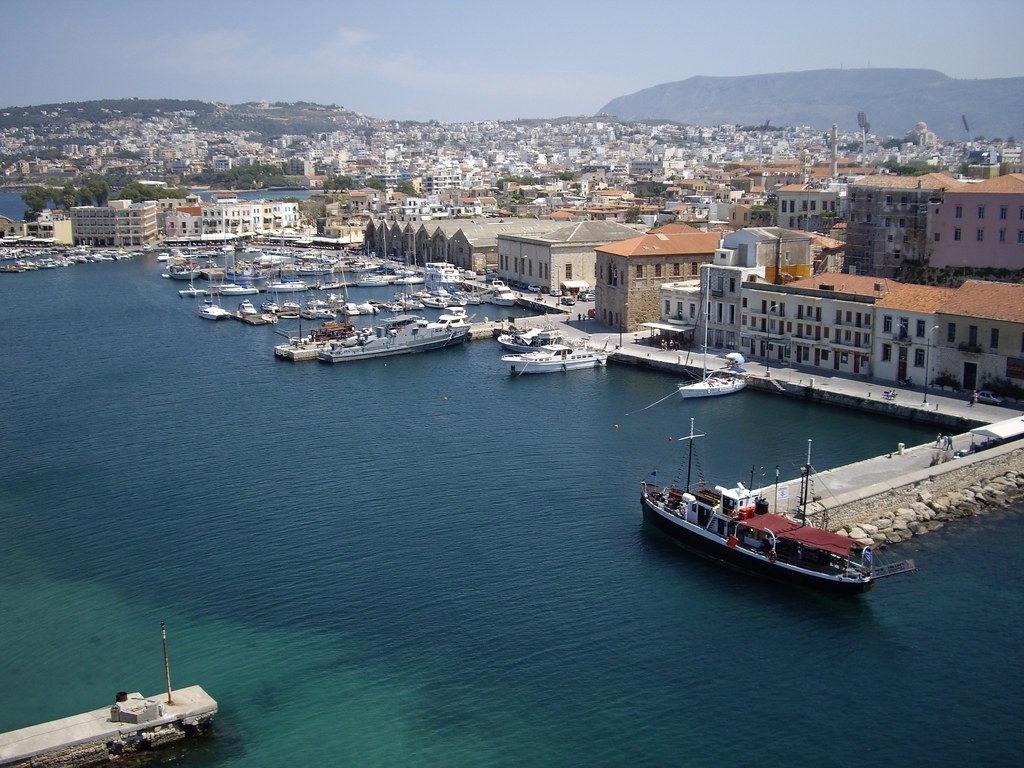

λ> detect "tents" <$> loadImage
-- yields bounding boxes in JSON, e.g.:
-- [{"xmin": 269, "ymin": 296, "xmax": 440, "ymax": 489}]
[
  {"xmin": 562, "ymin": 280, "xmax": 589, "ymax": 296},
  {"xmin": 639, "ymin": 320, "xmax": 695, "ymax": 347}
]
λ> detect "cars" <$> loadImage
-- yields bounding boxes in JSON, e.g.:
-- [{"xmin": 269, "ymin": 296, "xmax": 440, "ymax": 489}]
[
  {"xmin": 550, "ymin": 289, "xmax": 563, "ymax": 296},
  {"xmin": 582, "ymin": 294, "xmax": 595, "ymax": 301},
  {"xmin": 528, "ymin": 284, "xmax": 540, "ymax": 292},
  {"xmin": 561, "ymin": 297, "xmax": 576, "ymax": 306},
  {"xmin": 977, "ymin": 390, "xmax": 1006, "ymax": 406}
]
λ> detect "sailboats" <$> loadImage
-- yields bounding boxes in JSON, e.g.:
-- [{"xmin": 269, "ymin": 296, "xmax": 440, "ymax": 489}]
[{"xmin": 676, "ymin": 267, "xmax": 747, "ymax": 398}]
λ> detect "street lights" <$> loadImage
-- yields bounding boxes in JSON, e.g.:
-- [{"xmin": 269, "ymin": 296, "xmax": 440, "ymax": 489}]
[
  {"xmin": 519, "ymin": 255, "xmax": 527, "ymax": 296},
  {"xmin": 620, "ymin": 303, "xmax": 631, "ymax": 347},
  {"xmin": 766, "ymin": 304, "xmax": 779, "ymax": 373},
  {"xmin": 923, "ymin": 325, "xmax": 940, "ymax": 402}
]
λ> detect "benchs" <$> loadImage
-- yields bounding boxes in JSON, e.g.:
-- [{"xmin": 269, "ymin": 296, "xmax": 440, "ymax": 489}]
[{"xmin": 883, "ymin": 392, "xmax": 898, "ymax": 399}]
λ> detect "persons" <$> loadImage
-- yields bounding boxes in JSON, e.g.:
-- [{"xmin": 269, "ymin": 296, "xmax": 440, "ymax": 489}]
[
  {"xmin": 566, "ymin": 312, "xmax": 570, "ymax": 325},
  {"xmin": 572, "ymin": 290, "xmax": 580, "ymax": 301},
  {"xmin": 726, "ymin": 358, "xmax": 730, "ymax": 368},
  {"xmin": 935, "ymin": 433, "xmax": 953, "ymax": 451},
  {"xmin": 578, "ymin": 313, "xmax": 585, "ymax": 321},
  {"xmin": 650, "ymin": 333, "xmax": 684, "ymax": 351},
  {"xmin": 887, "ymin": 390, "xmax": 896, "ymax": 400},
  {"xmin": 969, "ymin": 390, "xmax": 977, "ymax": 407}
]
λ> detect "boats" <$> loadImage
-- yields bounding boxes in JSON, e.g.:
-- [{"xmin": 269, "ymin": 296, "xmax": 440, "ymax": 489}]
[
  {"xmin": 501, "ymin": 331, "xmax": 607, "ymax": 374},
  {"xmin": 289, "ymin": 298, "xmax": 355, "ymax": 347},
  {"xmin": 641, "ymin": 416, "xmax": 917, "ymax": 595},
  {"xmin": 0, "ymin": 234, "xmax": 517, "ymax": 332},
  {"xmin": 316, "ymin": 282, "xmax": 469, "ymax": 363},
  {"xmin": 498, "ymin": 311, "xmax": 563, "ymax": 353}
]
